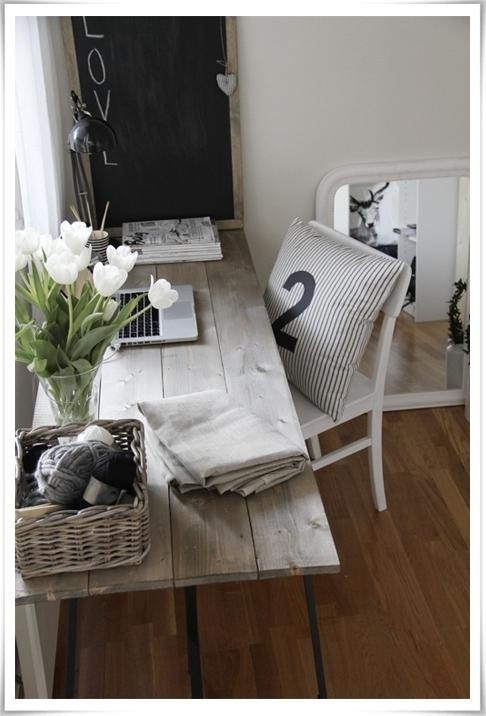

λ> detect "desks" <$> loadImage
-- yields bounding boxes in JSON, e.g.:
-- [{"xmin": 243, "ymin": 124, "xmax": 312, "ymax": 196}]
[{"xmin": 14, "ymin": 225, "xmax": 343, "ymax": 698}]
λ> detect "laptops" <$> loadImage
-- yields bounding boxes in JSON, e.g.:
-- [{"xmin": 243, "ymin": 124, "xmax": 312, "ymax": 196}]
[{"xmin": 50, "ymin": 235, "xmax": 200, "ymax": 348}]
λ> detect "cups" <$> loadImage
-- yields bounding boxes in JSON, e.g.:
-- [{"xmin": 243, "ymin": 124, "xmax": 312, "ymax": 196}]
[{"xmin": 85, "ymin": 230, "xmax": 109, "ymax": 266}]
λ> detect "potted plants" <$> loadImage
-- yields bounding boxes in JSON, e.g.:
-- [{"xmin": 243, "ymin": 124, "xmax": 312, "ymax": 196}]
[{"xmin": 446, "ymin": 277, "xmax": 468, "ymax": 389}]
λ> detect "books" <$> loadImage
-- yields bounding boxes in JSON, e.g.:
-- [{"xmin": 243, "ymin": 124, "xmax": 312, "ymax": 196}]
[{"xmin": 122, "ymin": 215, "xmax": 224, "ymax": 265}]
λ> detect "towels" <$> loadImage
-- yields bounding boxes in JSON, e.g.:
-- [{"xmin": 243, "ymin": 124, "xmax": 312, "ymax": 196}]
[{"xmin": 135, "ymin": 388, "xmax": 311, "ymax": 499}]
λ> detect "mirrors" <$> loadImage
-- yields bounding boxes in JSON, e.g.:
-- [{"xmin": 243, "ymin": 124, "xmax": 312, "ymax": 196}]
[{"xmin": 315, "ymin": 157, "xmax": 470, "ymax": 412}]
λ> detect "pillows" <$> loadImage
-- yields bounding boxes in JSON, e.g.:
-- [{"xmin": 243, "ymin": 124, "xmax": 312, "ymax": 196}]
[{"xmin": 262, "ymin": 214, "xmax": 406, "ymax": 422}]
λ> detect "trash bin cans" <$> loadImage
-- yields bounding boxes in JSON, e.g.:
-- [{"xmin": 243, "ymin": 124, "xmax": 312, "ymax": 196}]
[{"xmin": 393, "ymin": 223, "xmax": 416, "ymax": 303}]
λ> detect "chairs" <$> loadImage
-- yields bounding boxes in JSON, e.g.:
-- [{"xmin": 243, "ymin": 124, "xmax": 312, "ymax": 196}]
[{"xmin": 285, "ymin": 218, "xmax": 413, "ymax": 513}]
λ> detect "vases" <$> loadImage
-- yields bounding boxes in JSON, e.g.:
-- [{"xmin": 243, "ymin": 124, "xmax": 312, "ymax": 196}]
[{"xmin": 33, "ymin": 352, "xmax": 106, "ymax": 426}]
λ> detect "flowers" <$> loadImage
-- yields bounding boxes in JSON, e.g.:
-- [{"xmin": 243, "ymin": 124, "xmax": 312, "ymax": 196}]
[{"xmin": 14, "ymin": 219, "xmax": 181, "ymax": 426}]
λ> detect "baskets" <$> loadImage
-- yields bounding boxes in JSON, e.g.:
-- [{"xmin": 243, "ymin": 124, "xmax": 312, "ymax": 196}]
[{"xmin": 14, "ymin": 419, "xmax": 151, "ymax": 578}]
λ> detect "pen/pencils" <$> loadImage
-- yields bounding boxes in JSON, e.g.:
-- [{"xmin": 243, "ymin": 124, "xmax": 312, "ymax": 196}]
[{"xmin": 70, "ymin": 195, "xmax": 110, "ymax": 239}]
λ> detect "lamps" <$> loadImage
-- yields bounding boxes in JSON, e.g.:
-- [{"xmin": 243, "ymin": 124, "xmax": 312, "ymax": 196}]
[{"xmin": 67, "ymin": 89, "xmax": 124, "ymax": 229}]
